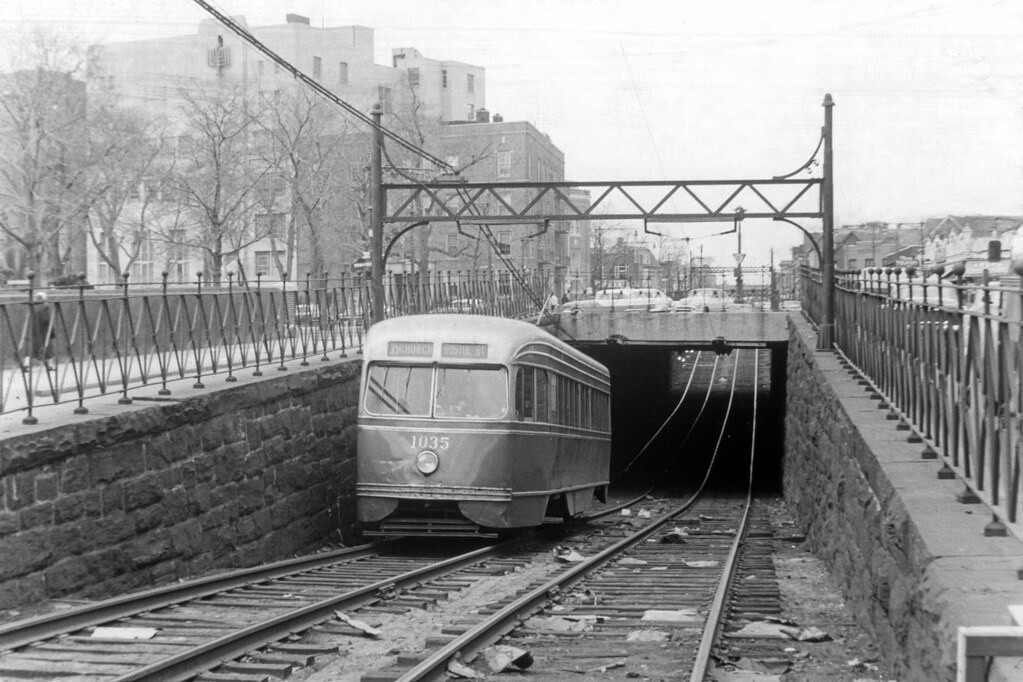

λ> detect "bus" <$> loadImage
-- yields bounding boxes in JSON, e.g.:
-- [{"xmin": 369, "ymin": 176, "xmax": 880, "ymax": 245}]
[{"xmin": 355, "ymin": 313, "xmax": 612, "ymax": 539}]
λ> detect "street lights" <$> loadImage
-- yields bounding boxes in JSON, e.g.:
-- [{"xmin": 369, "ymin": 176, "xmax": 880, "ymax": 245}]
[
  {"xmin": 733, "ymin": 206, "xmax": 745, "ymax": 304},
  {"xmin": 596, "ymin": 226, "xmax": 638, "ymax": 290}
]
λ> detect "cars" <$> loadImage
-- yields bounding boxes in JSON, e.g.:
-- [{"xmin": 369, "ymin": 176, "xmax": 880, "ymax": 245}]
[
  {"xmin": 295, "ymin": 304, "xmax": 320, "ymax": 320},
  {"xmin": 888, "ymin": 279, "xmax": 962, "ymax": 332},
  {"xmin": 671, "ymin": 288, "xmax": 733, "ymax": 312},
  {"xmin": 450, "ymin": 299, "xmax": 487, "ymax": 313},
  {"xmin": 858, "ymin": 266, "xmax": 906, "ymax": 294}
]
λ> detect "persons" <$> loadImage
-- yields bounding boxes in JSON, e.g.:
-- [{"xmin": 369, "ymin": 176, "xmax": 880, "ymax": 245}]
[
  {"xmin": 22, "ymin": 293, "xmax": 58, "ymax": 371},
  {"xmin": 551, "ymin": 290, "xmax": 570, "ymax": 311}
]
